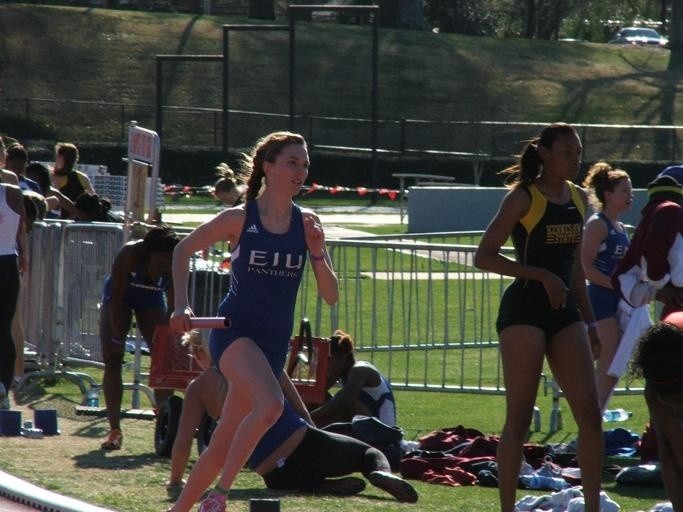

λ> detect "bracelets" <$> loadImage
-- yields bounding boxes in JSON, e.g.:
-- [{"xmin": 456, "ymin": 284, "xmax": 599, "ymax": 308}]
[{"xmin": 310, "ymin": 250, "xmax": 326, "ymax": 261}]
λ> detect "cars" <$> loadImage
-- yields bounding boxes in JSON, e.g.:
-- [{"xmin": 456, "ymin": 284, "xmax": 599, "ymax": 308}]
[
  {"xmin": 607, "ymin": 27, "xmax": 670, "ymax": 48},
  {"xmin": 309, "ymin": 0, "xmax": 379, "ymax": 25}
]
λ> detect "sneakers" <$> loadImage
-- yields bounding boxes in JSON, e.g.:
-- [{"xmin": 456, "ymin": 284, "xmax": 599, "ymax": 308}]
[
  {"xmin": 368, "ymin": 470, "xmax": 418, "ymax": 503},
  {"xmin": 102, "ymin": 430, "xmax": 122, "ymax": 449},
  {"xmin": 197, "ymin": 492, "xmax": 228, "ymax": 512},
  {"xmin": 317, "ymin": 476, "xmax": 366, "ymax": 496}
]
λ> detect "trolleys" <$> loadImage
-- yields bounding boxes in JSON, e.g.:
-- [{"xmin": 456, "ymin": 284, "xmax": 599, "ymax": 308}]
[{"xmin": 146, "ymin": 316, "xmax": 330, "ymax": 462}]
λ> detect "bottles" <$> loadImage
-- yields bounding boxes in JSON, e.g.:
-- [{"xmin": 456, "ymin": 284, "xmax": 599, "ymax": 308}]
[
  {"xmin": 602, "ymin": 408, "xmax": 634, "ymax": 422},
  {"xmin": 86, "ymin": 383, "xmax": 100, "ymax": 408}
]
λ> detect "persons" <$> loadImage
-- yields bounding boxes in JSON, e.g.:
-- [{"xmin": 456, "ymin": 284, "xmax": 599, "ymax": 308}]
[
  {"xmin": 170, "ymin": 131, "xmax": 341, "ymax": 511},
  {"xmin": 582, "ymin": 160, "xmax": 683, "ymax": 510},
  {"xmin": 209, "ymin": 169, "xmax": 245, "ymax": 207},
  {"xmin": 0, "ymin": 132, "xmax": 122, "ymax": 410},
  {"xmin": 99, "ymin": 225, "xmax": 181, "ymax": 450},
  {"xmin": 181, "ymin": 330, "xmax": 419, "ymax": 502},
  {"xmin": 472, "ymin": 126, "xmax": 606, "ymax": 512},
  {"xmin": 308, "ymin": 330, "xmax": 396, "ymax": 428}
]
